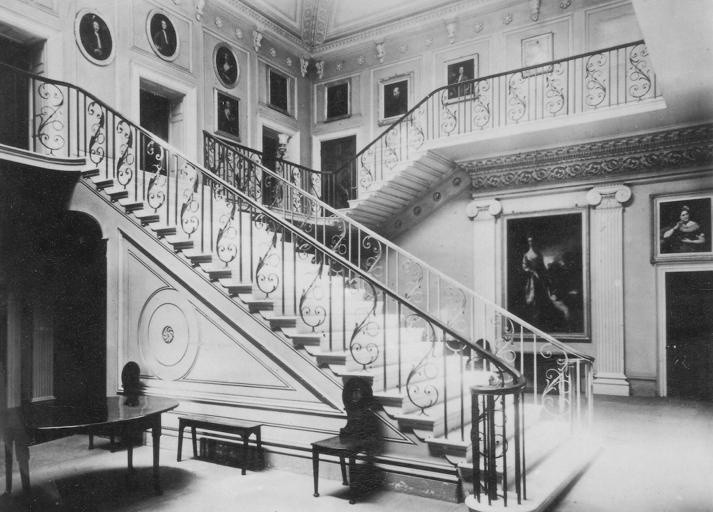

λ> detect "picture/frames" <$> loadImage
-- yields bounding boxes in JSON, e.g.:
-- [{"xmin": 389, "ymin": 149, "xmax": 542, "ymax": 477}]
[
  {"xmin": 145, "ymin": 9, "xmax": 181, "ymax": 61},
  {"xmin": 379, "ymin": 71, "xmax": 413, "ymax": 126},
  {"xmin": 213, "ymin": 86, "xmax": 242, "ymax": 142},
  {"xmin": 495, "ymin": 203, "xmax": 593, "ymax": 344},
  {"xmin": 74, "ymin": 8, "xmax": 116, "ymax": 65},
  {"xmin": 265, "ymin": 65, "xmax": 292, "ymax": 117},
  {"xmin": 519, "ymin": 31, "xmax": 556, "ymax": 78},
  {"xmin": 323, "ymin": 77, "xmax": 352, "ymax": 122},
  {"xmin": 649, "ymin": 185, "xmax": 713, "ymax": 264},
  {"xmin": 212, "ymin": 42, "xmax": 241, "ymax": 88},
  {"xmin": 441, "ymin": 53, "xmax": 479, "ymax": 104}
]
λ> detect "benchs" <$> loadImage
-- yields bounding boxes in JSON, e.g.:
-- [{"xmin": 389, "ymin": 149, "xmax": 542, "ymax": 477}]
[{"xmin": 177, "ymin": 413, "xmax": 266, "ymax": 474}]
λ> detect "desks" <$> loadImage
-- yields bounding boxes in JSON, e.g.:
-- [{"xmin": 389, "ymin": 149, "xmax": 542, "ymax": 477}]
[{"xmin": 0, "ymin": 393, "xmax": 176, "ymax": 512}]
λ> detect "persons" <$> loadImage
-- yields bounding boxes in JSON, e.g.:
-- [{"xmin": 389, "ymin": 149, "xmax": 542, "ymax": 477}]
[
  {"xmin": 219, "ymin": 99, "xmax": 238, "ymax": 132},
  {"xmin": 217, "ymin": 50, "xmax": 234, "ymax": 85},
  {"xmin": 150, "ymin": 20, "xmax": 173, "ymax": 54},
  {"xmin": 449, "ymin": 64, "xmax": 470, "ymax": 98},
  {"xmin": 663, "ymin": 204, "xmax": 707, "ymax": 252},
  {"xmin": 385, "ymin": 84, "xmax": 405, "ymax": 114},
  {"xmin": 80, "ymin": 19, "xmax": 112, "ymax": 60},
  {"xmin": 522, "ymin": 232, "xmax": 570, "ymax": 333}
]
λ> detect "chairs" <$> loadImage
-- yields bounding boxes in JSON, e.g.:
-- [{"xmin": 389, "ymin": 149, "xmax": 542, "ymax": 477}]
[
  {"xmin": 88, "ymin": 366, "xmax": 138, "ymax": 452},
  {"xmin": 313, "ymin": 383, "xmax": 382, "ymax": 504}
]
